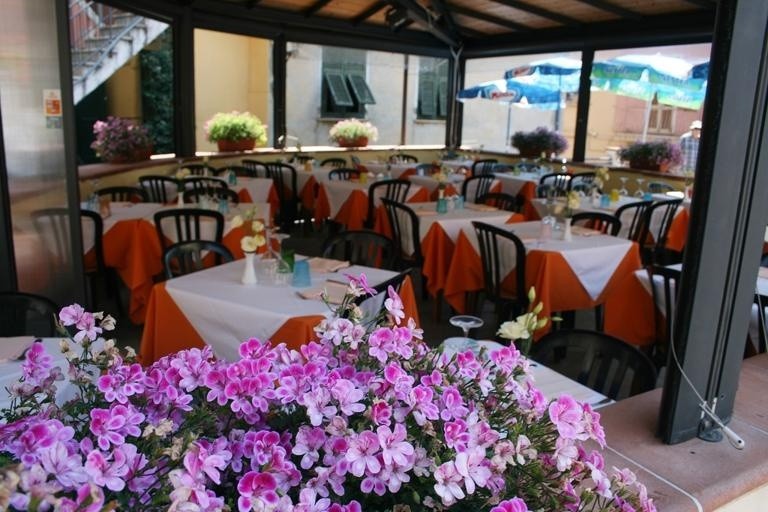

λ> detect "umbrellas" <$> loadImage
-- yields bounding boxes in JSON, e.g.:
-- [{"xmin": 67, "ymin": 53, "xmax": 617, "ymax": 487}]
[
  {"xmin": 454, "ymin": 77, "xmax": 565, "ymax": 151},
  {"xmin": 679, "ymin": 59, "xmax": 708, "ymax": 88},
  {"xmin": 503, "ymin": 58, "xmax": 622, "ymax": 148},
  {"xmin": 581, "ymin": 51, "xmax": 705, "ymax": 168}
]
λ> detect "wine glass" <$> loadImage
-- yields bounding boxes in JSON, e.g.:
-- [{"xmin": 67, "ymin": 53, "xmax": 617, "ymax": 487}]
[
  {"xmin": 447, "ymin": 314, "xmax": 485, "ymax": 338},
  {"xmin": 619, "ymin": 176, "xmax": 644, "ymax": 197}
]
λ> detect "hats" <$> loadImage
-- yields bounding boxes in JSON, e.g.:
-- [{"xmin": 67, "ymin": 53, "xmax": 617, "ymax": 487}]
[{"xmin": 690, "ymin": 121, "xmax": 703, "ymax": 129}]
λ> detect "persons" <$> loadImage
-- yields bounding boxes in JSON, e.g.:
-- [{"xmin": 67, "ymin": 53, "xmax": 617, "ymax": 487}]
[{"xmin": 676, "ymin": 121, "xmax": 701, "ymax": 177}]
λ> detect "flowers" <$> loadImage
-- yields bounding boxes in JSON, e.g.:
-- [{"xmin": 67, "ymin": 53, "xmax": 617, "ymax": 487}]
[
  {"xmin": 205, "ymin": 110, "xmax": 265, "ymax": 143},
  {"xmin": 511, "ymin": 128, "xmax": 567, "ymax": 154},
  {"xmin": 617, "ymin": 140, "xmax": 687, "ymax": 166},
  {"xmin": 0, "ymin": 274, "xmax": 654, "ymax": 510},
  {"xmin": 90, "ymin": 118, "xmax": 155, "ymax": 153},
  {"xmin": 328, "ymin": 118, "xmax": 378, "ymax": 137}
]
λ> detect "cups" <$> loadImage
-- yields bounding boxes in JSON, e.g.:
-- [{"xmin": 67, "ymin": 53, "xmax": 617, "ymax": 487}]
[
  {"xmin": 290, "ymin": 260, "xmax": 311, "ymax": 287},
  {"xmin": 305, "ymin": 159, "xmax": 314, "ymax": 172},
  {"xmin": 277, "ymin": 237, "xmax": 298, "ymax": 273},
  {"xmin": 601, "ymin": 195, "xmax": 611, "ymax": 208},
  {"xmin": 539, "ymin": 222, "xmax": 553, "ymax": 241},
  {"xmin": 610, "ymin": 189, "xmax": 619, "ymax": 201},
  {"xmin": 359, "ymin": 172, "xmax": 368, "ymax": 186},
  {"xmin": 453, "ymin": 195, "xmax": 465, "ymax": 212}
]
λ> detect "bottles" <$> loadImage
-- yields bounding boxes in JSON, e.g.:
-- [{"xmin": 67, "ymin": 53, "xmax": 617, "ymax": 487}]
[
  {"xmin": 384, "ymin": 165, "xmax": 394, "ymax": 181},
  {"xmin": 539, "ymin": 150, "xmax": 568, "ymax": 174}
]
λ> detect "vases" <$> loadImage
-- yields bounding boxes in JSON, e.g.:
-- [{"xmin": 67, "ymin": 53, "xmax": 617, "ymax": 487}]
[
  {"xmin": 109, "ymin": 145, "xmax": 152, "ymax": 162},
  {"xmin": 630, "ymin": 157, "xmax": 670, "ymax": 173},
  {"xmin": 338, "ymin": 135, "xmax": 369, "ymax": 147},
  {"xmin": 217, "ymin": 137, "xmax": 255, "ymax": 150},
  {"xmin": 520, "ymin": 147, "xmax": 555, "ymax": 159}
]
